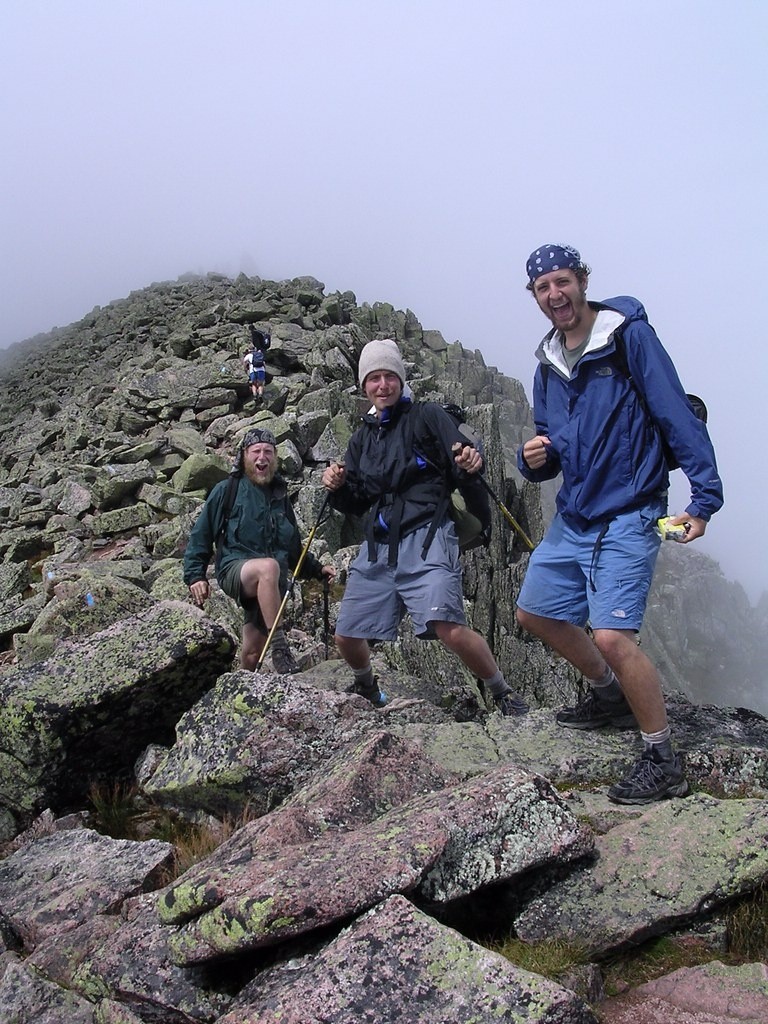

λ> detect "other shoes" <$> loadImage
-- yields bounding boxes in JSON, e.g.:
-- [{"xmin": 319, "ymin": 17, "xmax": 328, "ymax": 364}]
[{"xmin": 254, "ymin": 395, "xmax": 263, "ymax": 404}]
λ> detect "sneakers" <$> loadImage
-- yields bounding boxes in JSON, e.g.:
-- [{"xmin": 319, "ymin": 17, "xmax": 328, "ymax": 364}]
[
  {"xmin": 493, "ymin": 689, "xmax": 530, "ymax": 716},
  {"xmin": 272, "ymin": 647, "xmax": 302, "ymax": 674},
  {"xmin": 556, "ymin": 681, "xmax": 638, "ymax": 729},
  {"xmin": 343, "ymin": 674, "xmax": 381, "ymax": 702},
  {"xmin": 608, "ymin": 742, "xmax": 689, "ymax": 805}
]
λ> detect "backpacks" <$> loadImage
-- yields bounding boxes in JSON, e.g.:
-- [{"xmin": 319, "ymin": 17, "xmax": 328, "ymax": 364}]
[
  {"xmin": 357, "ymin": 403, "xmax": 492, "ymax": 552},
  {"xmin": 255, "ymin": 330, "xmax": 271, "ymax": 348},
  {"xmin": 250, "ymin": 351, "xmax": 264, "ymax": 366},
  {"xmin": 614, "ymin": 317, "xmax": 707, "ymax": 472}
]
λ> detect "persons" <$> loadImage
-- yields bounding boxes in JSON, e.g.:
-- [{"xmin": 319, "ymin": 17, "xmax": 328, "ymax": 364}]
[
  {"xmin": 321, "ymin": 339, "xmax": 530, "ymax": 718},
  {"xmin": 248, "ymin": 325, "xmax": 266, "ymax": 353},
  {"xmin": 183, "ymin": 429, "xmax": 337, "ymax": 674},
  {"xmin": 244, "ymin": 347, "xmax": 265, "ymax": 404},
  {"xmin": 516, "ymin": 244, "xmax": 723, "ymax": 802}
]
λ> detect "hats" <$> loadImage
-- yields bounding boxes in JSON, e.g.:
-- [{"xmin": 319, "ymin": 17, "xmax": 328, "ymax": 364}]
[{"xmin": 358, "ymin": 338, "xmax": 405, "ymax": 390}]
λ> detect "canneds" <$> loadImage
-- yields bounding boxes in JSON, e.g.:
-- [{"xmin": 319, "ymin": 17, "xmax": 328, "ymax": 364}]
[{"xmin": 265, "ymin": 339, "xmax": 268, "ymax": 344}]
